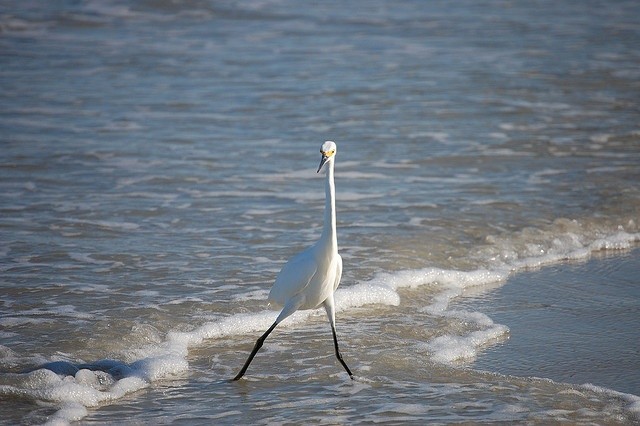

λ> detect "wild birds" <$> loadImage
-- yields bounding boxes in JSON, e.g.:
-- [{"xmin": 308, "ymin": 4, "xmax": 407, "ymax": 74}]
[{"xmin": 231, "ymin": 139, "xmax": 358, "ymax": 383}]
